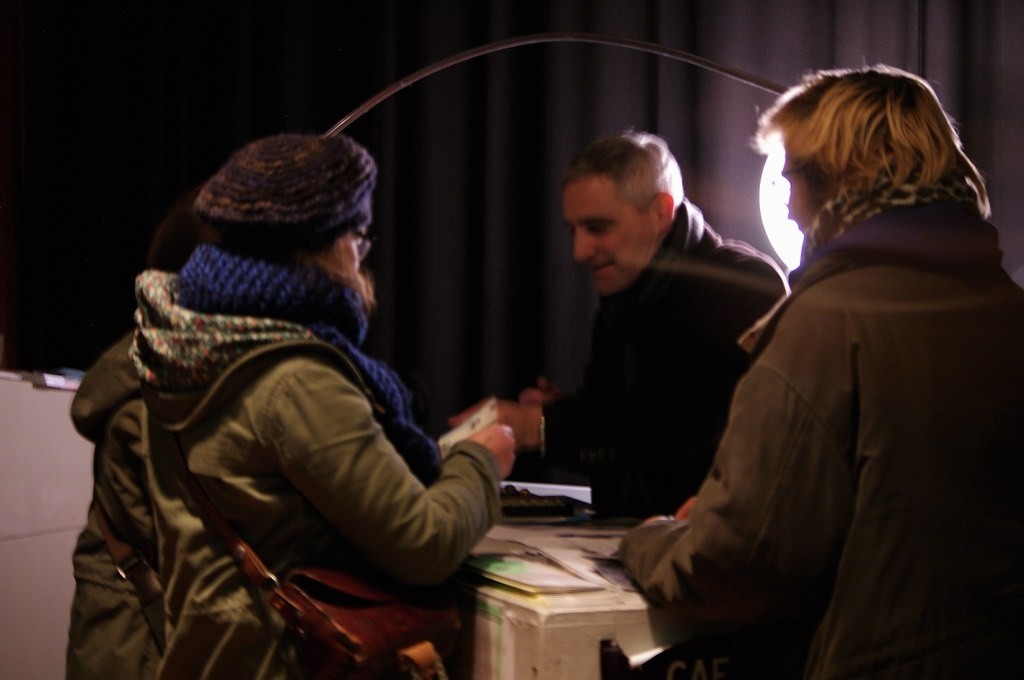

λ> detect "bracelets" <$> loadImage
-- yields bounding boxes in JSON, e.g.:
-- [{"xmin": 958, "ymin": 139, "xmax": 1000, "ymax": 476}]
[{"xmin": 541, "ymin": 416, "xmax": 545, "ymax": 452}]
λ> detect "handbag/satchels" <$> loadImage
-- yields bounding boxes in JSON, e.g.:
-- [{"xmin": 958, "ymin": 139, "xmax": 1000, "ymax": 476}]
[{"xmin": 276, "ymin": 565, "xmax": 461, "ymax": 680}]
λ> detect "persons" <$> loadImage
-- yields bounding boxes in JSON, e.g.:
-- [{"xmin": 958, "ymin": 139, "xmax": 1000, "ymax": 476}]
[
  {"xmin": 620, "ymin": 68, "xmax": 1024, "ymax": 680},
  {"xmin": 65, "ymin": 134, "xmax": 515, "ymax": 680},
  {"xmin": 448, "ymin": 130, "xmax": 790, "ymax": 523}
]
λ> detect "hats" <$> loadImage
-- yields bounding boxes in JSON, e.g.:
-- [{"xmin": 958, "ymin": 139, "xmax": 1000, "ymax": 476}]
[{"xmin": 188, "ymin": 135, "xmax": 378, "ymax": 246}]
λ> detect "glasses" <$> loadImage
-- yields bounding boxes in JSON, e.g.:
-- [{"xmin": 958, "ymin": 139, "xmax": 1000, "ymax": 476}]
[{"xmin": 348, "ymin": 230, "xmax": 372, "ymax": 258}]
